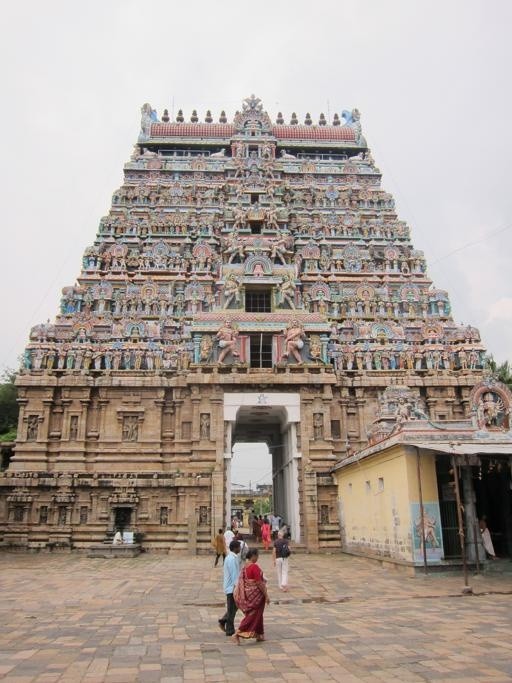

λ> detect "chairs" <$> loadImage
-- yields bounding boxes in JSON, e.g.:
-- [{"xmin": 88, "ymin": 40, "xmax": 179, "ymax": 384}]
[
  {"xmin": 256, "ymin": 637, "xmax": 264, "ymax": 642},
  {"xmin": 218, "ymin": 618, "xmax": 242, "ymax": 647},
  {"xmin": 279, "ymin": 586, "xmax": 289, "ymax": 592}
]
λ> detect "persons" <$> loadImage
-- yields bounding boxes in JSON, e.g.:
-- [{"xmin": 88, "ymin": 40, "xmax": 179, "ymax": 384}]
[
  {"xmin": 224, "ymin": 233, "xmax": 245, "ymax": 265},
  {"xmin": 161, "ymin": 508, "xmax": 168, "ymax": 525},
  {"xmin": 222, "ymin": 273, "xmax": 241, "ymax": 309},
  {"xmin": 201, "ymin": 414, "xmax": 209, "ymax": 438},
  {"xmin": 200, "ymin": 507, "xmax": 207, "ymax": 524},
  {"xmin": 235, "ymin": 143, "xmax": 275, "ymax": 179},
  {"xmin": 483, "ymin": 393, "xmax": 505, "ymax": 427},
  {"xmin": 236, "ymin": 184, "xmax": 246, "ymax": 201},
  {"xmin": 217, "ymin": 318, "xmax": 244, "ymax": 364},
  {"xmin": 395, "ymin": 396, "xmax": 413, "ymax": 423},
  {"xmin": 14, "ymin": 506, "xmax": 23, "ymax": 521},
  {"xmin": 282, "ymin": 319, "xmax": 304, "ymax": 366},
  {"xmin": 58, "ymin": 507, "xmax": 66, "ymax": 526},
  {"xmin": 270, "ymin": 232, "xmax": 293, "ymax": 268},
  {"xmin": 31, "ymin": 153, "xmax": 225, "ymax": 366},
  {"xmin": 314, "ymin": 414, "xmax": 323, "ymax": 440},
  {"xmin": 266, "ymin": 203, "xmax": 279, "ymax": 230},
  {"xmin": 265, "ymin": 180, "xmax": 274, "ymax": 199},
  {"xmin": 27, "ymin": 417, "xmax": 38, "ymax": 439},
  {"xmin": 213, "ymin": 510, "xmax": 292, "ymax": 646},
  {"xmin": 232, "ymin": 202, "xmax": 246, "ymax": 231},
  {"xmin": 41, "ymin": 506, "xmax": 47, "ymax": 523},
  {"xmin": 278, "ymin": 156, "xmax": 492, "ymax": 371},
  {"xmin": 322, "ymin": 506, "xmax": 328, "ymax": 525},
  {"xmin": 80, "ymin": 508, "xmax": 87, "ymax": 524},
  {"xmin": 123, "ymin": 416, "xmax": 137, "ymax": 441},
  {"xmin": 415, "ymin": 507, "xmax": 439, "ymax": 556},
  {"xmin": 479, "ymin": 513, "xmax": 499, "ymax": 560},
  {"xmin": 277, "ymin": 272, "xmax": 296, "ymax": 309},
  {"xmin": 365, "ymin": 415, "xmax": 374, "ymax": 447}
]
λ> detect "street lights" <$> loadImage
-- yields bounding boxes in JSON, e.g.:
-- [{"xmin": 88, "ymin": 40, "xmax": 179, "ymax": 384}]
[
  {"xmin": 282, "ymin": 539, "xmax": 292, "ymax": 558},
  {"xmin": 241, "ymin": 540, "xmax": 247, "ymax": 561}
]
